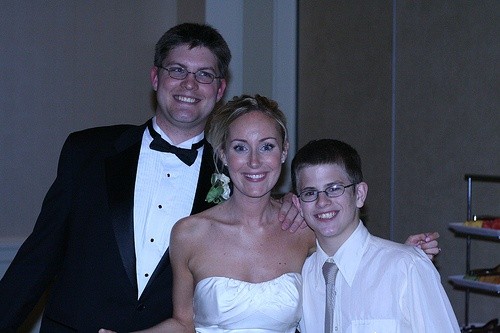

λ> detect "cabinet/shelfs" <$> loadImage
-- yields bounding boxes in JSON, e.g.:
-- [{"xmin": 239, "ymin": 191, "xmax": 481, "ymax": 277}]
[{"xmin": 448, "ymin": 173, "xmax": 500, "ymax": 333}]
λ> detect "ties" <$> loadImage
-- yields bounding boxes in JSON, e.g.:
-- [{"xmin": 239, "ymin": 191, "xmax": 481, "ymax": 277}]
[{"xmin": 321, "ymin": 262, "xmax": 339, "ymax": 333}]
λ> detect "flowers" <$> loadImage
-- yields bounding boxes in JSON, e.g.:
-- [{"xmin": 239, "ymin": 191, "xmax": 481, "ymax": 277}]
[{"xmin": 204, "ymin": 173, "xmax": 231, "ymax": 205}]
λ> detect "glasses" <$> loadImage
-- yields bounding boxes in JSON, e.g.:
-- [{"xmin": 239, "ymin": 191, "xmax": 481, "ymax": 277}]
[
  {"xmin": 296, "ymin": 182, "xmax": 358, "ymax": 202},
  {"xmin": 154, "ymin": 63, "xmax": 225, "ymax": 84}
]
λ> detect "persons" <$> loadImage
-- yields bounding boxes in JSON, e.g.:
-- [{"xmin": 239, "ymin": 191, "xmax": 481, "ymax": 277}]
[
  {"xmin": 99, "ymin": 94, "xmax": 440, "ymax": 333},
  {"xmin": 291, "ymin": 139, "xmax": 461, "ymax": 333},
  {"xmin": 0, "ymin": 23, "xmax": 308, "ymax": 333}
]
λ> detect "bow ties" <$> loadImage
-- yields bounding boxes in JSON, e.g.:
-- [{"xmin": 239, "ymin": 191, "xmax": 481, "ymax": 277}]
[{"xmin": 147, "ymin": 118, "xmax": 207, "ymax": 166}]
